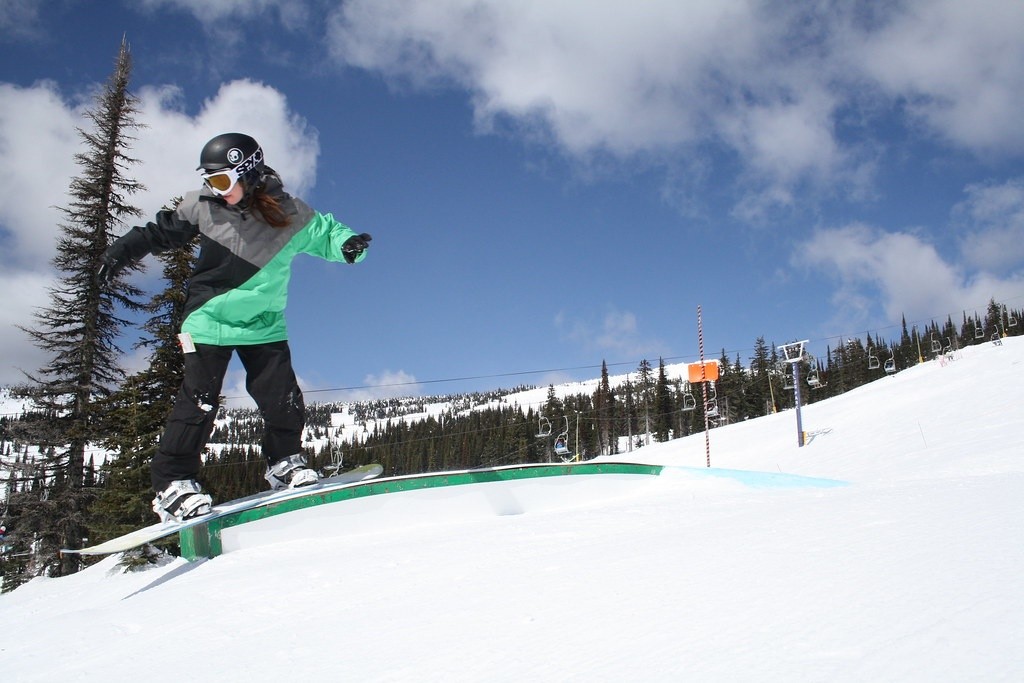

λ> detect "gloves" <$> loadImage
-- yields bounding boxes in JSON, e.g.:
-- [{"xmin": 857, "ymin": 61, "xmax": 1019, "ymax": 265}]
[
  {"xmin": 341, "ymin": 232, "xmax": 372, "ymax": 264},
  {"xmin": 92, "ymin": 252, "xmax": 123, "ymax": 289}
]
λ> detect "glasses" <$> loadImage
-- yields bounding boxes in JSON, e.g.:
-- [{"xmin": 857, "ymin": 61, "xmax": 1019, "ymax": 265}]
[{"xmin": 200, "ymin": 146, "xmax": 263, "ymax": 196}]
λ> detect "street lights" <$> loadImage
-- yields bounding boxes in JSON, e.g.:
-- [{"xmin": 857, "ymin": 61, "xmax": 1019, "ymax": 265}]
[{"xmin": 777, "ymin": 340, "xmax": 810, "ymax": 445}]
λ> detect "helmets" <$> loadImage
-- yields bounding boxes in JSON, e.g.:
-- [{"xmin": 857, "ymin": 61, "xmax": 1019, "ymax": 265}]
[{"xmin": 195, "ymin": 131, "xmax": 265, "ymax": 176}]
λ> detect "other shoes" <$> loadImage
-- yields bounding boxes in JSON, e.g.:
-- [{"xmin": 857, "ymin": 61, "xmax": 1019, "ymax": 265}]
[
  {"xmin": 267, "ymin": 464, "xmax": 318, "ymax": 486},
  {"xmin": 156, "ymin": 489, "xmax": 212, "ymax": 518}
]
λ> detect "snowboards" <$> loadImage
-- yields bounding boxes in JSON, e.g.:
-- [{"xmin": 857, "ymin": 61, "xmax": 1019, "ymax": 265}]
[{"xmin": 79, "ymin": 463, "xmax": 384, "ymax": 555}]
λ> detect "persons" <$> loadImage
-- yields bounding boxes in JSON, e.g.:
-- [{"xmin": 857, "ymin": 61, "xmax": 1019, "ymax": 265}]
[{"xmin": 95, "ymin": 133, "xmax": 371, "ymax": 522}]
